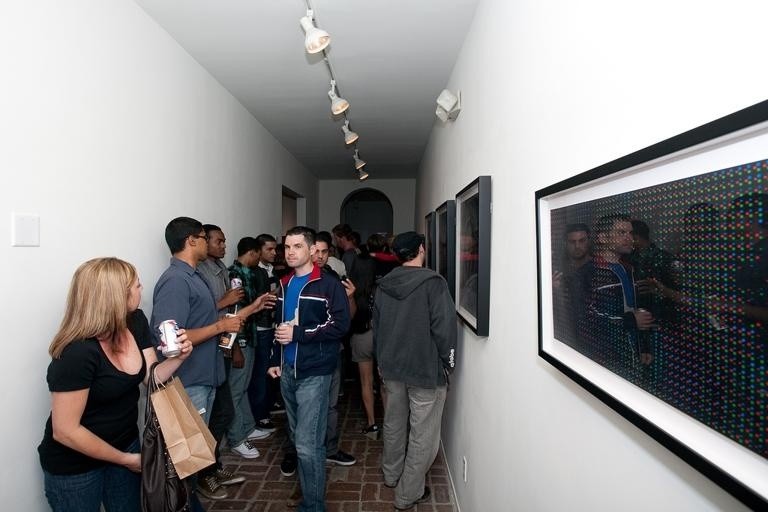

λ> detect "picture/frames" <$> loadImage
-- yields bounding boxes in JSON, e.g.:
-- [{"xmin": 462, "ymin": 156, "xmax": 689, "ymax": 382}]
[
  {"xmin": 436, "ymin": 200, "xmax": 456, "ymax": 306},
  {"xmin": 452, "ymin": 176, "xmax": 491, "ymax": 338},
  {"xmin": 425, "ymin": 211, "xmax": 436, "ymax": 271},
  {"xmin": 533, "ymin": 99, "xmax": 768, "ymax": 512}
]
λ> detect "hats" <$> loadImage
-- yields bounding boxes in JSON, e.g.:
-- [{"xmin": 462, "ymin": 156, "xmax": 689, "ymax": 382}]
[{"xmin": 392, "ymin": 231, "xmax": 422, "ymax": 256}]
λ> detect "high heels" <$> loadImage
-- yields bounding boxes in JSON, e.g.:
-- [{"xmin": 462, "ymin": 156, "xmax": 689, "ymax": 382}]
[{"xmin": 362, "ymin": 424, "xmax": 378, "ymax": 440}]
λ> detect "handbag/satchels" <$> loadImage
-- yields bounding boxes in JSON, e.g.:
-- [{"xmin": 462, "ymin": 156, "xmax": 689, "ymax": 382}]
[
  {"xmin": 151, "ymin": 364, "xmax": 218, "ymax": 480},
  {"xmin": 141, "ymin": 362, "xmax": 205, "ymax": 511}
]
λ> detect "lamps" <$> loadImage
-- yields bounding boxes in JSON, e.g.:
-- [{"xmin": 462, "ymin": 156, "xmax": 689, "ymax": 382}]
[
  {"xmin": 299, "ymin": 0, "xmax": 367, "ymax": 180},
  {"xmin": 433, "ymin": 88, "xmax": 459, "ymax": 124}
]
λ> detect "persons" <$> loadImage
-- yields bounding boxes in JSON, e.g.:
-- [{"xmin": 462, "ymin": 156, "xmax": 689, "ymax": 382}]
[
  {"xmin": 265, "ymin": 225, "xmax": 354, "ymax": 512},
  {"xmin": 38, "ymin": 257, "xmax": 194, "ymax": 510},
  {"xmin": 374, "ymin": 232, "xmax": 457, "ymax": 510},
  {"xmin": 551, "ymin": 189, "xmax": 766, "ymax": 448},
  {"xmin": 459, "ymin": 232, "xmax": 478, "ymax": 272},
  {"xmin": 200, "ymin": 218, "xmax": 400, "ymax": 478},
  {"xmin": 152, "ymin": 215, "xmax": 277, "ymax": 502}
]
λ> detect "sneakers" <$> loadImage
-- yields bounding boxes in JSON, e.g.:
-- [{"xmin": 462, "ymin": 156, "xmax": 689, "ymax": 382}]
[
  {"xmin": 215, "ymin": 466, "xmax": 245, "ymax": 485},
  {"xmin": 326, "ymin": 449, "xmax": 356, "ymax": 465},
  {"xmin": 247, "ymin": 428, "xmax": 270, "ymax": 441},
  {"xmin": 395, "ymin": 485, "xmax": 431, "ymax": 510},
  {"xmin": 287, "ymin": 485, "xmax": 303, "ymax": 506},
  {"xmin": 269, "ymin": 402, "xmax": 286, "ymax": 414},
  {"xmin": 196, "ymin": 473, "xmax": 228, "ymax": 499},
  {"xmin": 385, "ymin": 483, "xmax": 396, "ymax": 488},
  {"xmin": 280, "ymin": 452, "xmax": 298, "ymax": 476},
  {"xmin": 231, "ymin": 441, "xmax": 260, "ymax": 458},
  {"xmin": 255, "ymin": 417, "xmax": 276, "ymax": 432}
]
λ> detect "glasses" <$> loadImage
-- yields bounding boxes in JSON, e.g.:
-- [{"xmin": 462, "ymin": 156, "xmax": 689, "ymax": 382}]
[{"xmin": 185, "ymin": 234, "xmax": 210, "ymax": 243}]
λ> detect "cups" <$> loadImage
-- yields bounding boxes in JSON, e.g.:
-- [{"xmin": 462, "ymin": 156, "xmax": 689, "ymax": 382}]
[{"xmin": 218, "ymin": 314, "xmax": 239, "ymax": 350}]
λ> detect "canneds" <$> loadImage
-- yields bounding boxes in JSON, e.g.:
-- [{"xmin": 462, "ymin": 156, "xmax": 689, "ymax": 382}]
[
  {"xmin": 157, "ymin": 319, "xmax": 182, "ymax": 359},
  {"xmin": 635, "ymin": 306, "xmax": 651, "ymax": 333},
  {"xmin": 278, "ymin": 322, "xmax": 290, "ymax": 345},
  {"xmin": 230, "ymin": 277, "xmax": 242, "ymax": 291}
]
